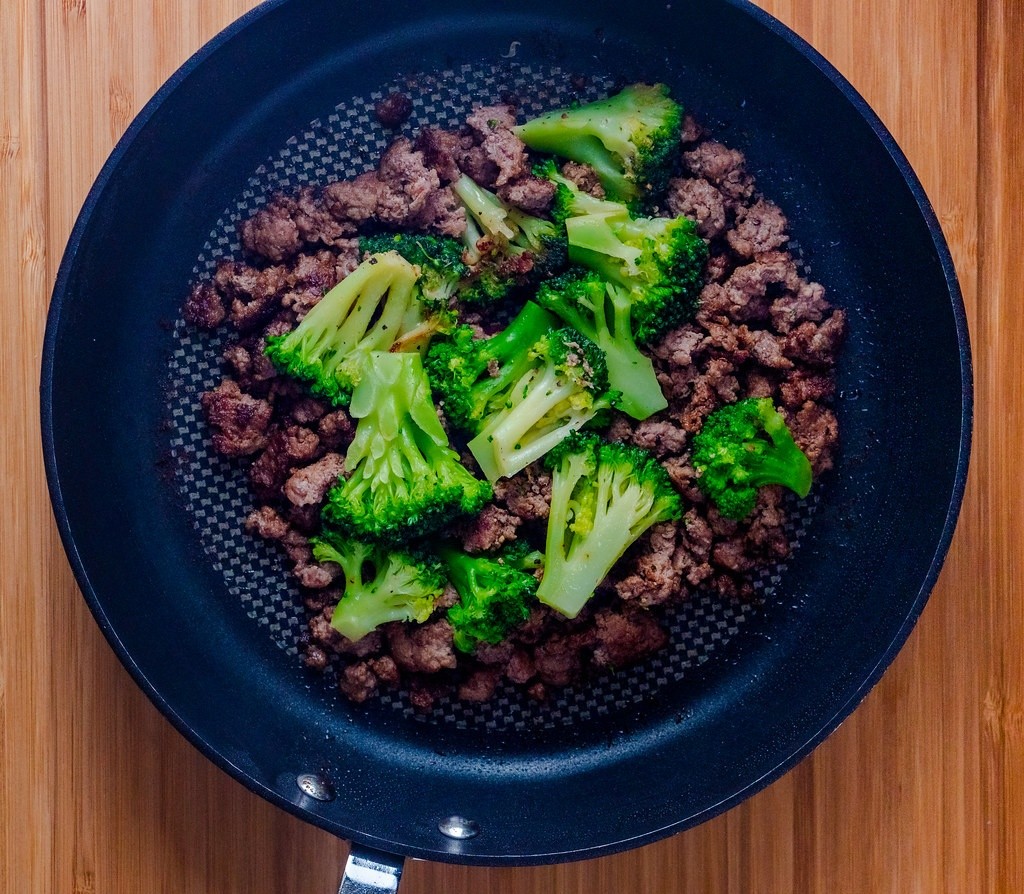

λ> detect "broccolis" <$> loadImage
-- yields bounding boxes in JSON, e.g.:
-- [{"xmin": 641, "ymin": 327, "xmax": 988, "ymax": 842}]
[{"xmin": 261, "ymin": 83, "xmax": 814, "ymax": 650}]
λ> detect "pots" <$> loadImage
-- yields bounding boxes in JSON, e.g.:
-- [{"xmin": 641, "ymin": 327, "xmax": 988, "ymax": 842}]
[{"xmin": 42, "ymin": 4, "xmax": 970, "ymax": 894}]
